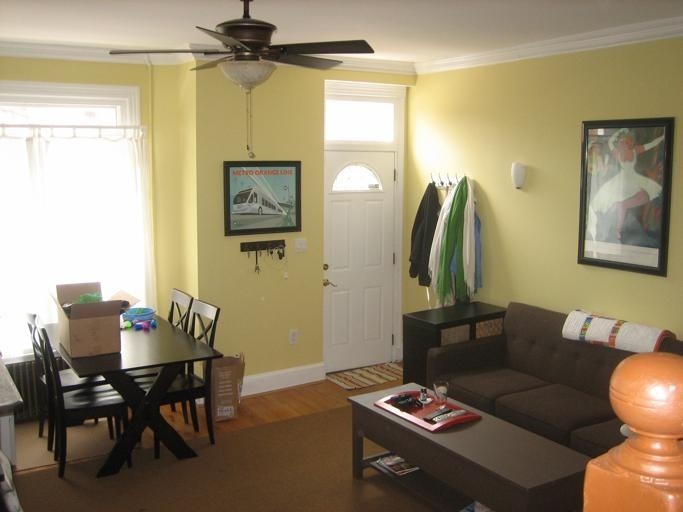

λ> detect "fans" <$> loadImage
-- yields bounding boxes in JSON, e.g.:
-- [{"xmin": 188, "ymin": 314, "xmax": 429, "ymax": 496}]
[{"xmin": 108, "ymin": 0, "xmax": 374, "ymax": 75}]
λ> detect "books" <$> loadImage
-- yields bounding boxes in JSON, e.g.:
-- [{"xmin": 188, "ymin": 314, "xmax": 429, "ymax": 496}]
[
  {"xmin": 368, "ymin": 453, "xmax": 418, "ymax": 478},
  {"xmin": 456, "ymin": 499, "xmax": 490, "ymax": 512}
]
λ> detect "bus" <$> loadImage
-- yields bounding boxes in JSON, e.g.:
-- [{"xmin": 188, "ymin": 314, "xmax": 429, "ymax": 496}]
[{"xmin": 231, "ymin": 186, "xmax": 284, "ymax": 215}]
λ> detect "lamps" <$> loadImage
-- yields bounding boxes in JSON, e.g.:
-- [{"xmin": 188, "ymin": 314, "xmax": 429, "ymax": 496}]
[
  {"xmin": 510, "ymin": 161, "xmax": 527, "ymax": 190},
  {"xmin": 217, "ymin": 57, "xmax": 275, "ymax": 161}
]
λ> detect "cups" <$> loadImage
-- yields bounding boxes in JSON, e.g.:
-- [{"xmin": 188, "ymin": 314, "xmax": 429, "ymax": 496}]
[{"xmin": 431, "ymin": 381, "xmax": 449, "ymax": 410}]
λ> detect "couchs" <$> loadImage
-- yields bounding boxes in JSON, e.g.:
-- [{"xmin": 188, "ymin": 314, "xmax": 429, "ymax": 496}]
[{"xmin": 425, "ymin": 301, "xmax": 681, "ymax": 459}]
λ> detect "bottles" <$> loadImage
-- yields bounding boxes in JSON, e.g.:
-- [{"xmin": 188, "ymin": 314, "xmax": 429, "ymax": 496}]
[{"xmin": 420, "ymin": 389, "xmax": 426, "ymax": 402}]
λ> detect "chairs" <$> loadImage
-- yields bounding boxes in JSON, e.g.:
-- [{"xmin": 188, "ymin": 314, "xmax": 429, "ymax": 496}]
[{"xmin": 24, "ymin": 288, "xmax": 223, "ymax": 478}]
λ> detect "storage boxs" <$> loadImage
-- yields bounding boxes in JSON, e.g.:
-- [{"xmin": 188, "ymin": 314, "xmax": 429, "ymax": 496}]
[{"xmin": 48, "ymin": 281, "xmax": 140, "ymax": 359}]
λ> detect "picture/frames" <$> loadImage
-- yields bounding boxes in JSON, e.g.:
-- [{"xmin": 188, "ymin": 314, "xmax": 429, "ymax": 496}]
[
  {"xmin": 576, "ymin": 115, "xmax": 676, "ymax": 280},
  {"xmin": 223, "ymin": 158, "xmax": 302, "ymax": 237}
]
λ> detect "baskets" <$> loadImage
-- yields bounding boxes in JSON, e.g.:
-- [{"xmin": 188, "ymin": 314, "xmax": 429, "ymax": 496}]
[{"xmin": 122, "ymin": 307, "xmax": 155, "ymax": 321}]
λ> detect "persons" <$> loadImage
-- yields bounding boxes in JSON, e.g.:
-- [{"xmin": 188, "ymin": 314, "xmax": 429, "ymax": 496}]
[{"xmin": 593, "ymin": 129, "xmax": 664, "ymax": 243}]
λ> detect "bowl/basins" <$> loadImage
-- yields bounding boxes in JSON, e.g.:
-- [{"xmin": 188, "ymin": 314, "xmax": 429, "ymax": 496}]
[{"xmin": 121, "ymin": 307, "xmax": 156, "ymax": 323}]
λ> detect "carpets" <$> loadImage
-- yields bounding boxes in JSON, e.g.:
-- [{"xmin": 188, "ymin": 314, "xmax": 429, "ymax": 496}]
[
  {"xmin": 7, "ymin": 405, "xmax": 492, "ymax": 512},
  {"xmin": 324, "ymin": 362, "xmax": 403, "ymax": 390}
]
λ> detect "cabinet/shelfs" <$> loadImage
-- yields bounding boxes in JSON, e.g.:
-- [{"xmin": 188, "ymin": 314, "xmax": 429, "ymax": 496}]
[{"xmin": 401, "ymin": 301, "xmax": 506, "ymax": 385}]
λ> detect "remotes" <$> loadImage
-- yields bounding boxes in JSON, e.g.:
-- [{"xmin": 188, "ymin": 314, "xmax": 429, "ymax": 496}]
[
  {"xmin": 433, "ymin": 409, "xmax": 466, "ymax": 423},
  {"xmin": 424, "ymin": 406, "xmax": 450, "ymax": 420}
]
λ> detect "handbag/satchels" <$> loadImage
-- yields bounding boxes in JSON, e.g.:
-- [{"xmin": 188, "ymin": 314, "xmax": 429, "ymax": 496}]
[{"xmin": 202, "ymin": 352, "xmax": 244, "ymax": 422}]
